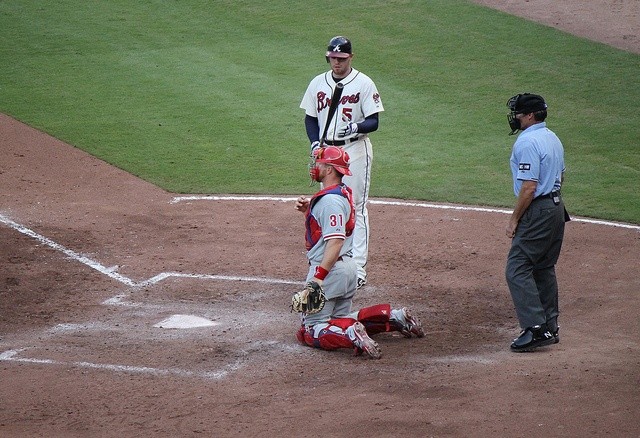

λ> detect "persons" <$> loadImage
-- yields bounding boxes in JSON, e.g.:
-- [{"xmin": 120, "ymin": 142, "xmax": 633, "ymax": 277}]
[
  {"xmin": 291, "ymin": 144, "xmax": 425, "ymax": 361},
  {"xmin": 303, "ymin": 34, "xmax": 379, "ymax": 293},
  {"xmin": 503, "ymin": 91, "xmax": 571, "ymax": 352}
]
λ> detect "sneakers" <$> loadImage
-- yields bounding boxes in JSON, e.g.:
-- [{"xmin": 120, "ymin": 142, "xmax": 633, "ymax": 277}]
[
  {"xmin": 347, "ymin": 322, "xmax": 382, "ymax": 359},
  {"xmin": 512, "ymin": 325, "xmax": 555, "ymax": 351},
  {"xmin": 545, "ymin": 329, "xmax": 560, "ymax": 345},
  {"xmin": 392, "ymin": 306, "xmax": 425, "ymax": 337}
]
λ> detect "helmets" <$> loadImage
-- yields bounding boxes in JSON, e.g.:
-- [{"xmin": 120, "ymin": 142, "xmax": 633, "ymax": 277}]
[
  {"xmin": 507, "ymin": 94, "xmax": 544, "ymax": 130},
  {"xmin": 312, "ymin": 146, "xmax": 352, "ymax": 178},
  {"xmin": 325, "ymin": 36, "xmax": 352, "ymax": 63}
]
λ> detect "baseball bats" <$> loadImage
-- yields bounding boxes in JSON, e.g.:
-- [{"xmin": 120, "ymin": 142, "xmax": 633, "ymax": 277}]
[{"xmin": 319, "ymin": 82, "xmax": 345, "ymax": 146}]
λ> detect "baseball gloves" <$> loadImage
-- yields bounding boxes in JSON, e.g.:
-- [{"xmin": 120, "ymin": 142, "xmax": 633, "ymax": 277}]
[{"xmin": 291, "ymin": 281, "xmax": 326, "ymax": 314}]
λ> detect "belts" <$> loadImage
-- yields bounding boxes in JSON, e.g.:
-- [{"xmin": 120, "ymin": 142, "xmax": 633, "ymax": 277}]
[{"xmin": 535, "ymin": 188, "xmax": 563, "ymax": 200}]
